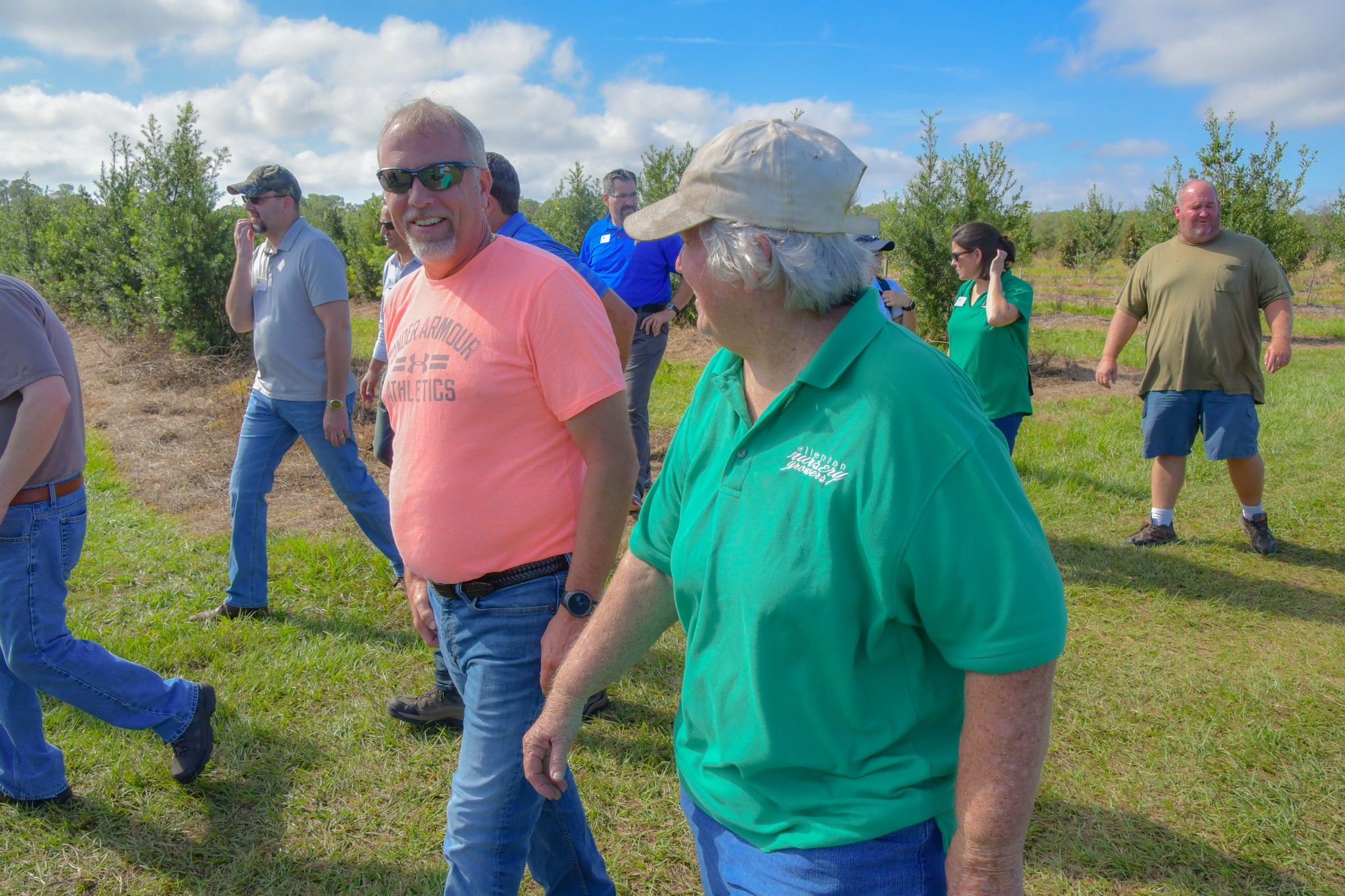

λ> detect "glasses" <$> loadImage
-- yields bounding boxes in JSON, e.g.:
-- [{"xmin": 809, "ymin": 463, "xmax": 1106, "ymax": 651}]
[
  {"xmin": 608, "ymin": 193, "xmax": 640, "ymax": 199},
  {"xmin": 241, "ymin": 194, "xmax": 286, "ymax": 205},
  {"xmin": 376, "ymin": 161, "xmax": 483, "ymax": 194},
  {"xmin": 379, "ymin": 218, "xmax": 395, "ymax": 230},
  {"xmin": 951, "ymin": 247, "xmax": 976, "ymax": 262}
]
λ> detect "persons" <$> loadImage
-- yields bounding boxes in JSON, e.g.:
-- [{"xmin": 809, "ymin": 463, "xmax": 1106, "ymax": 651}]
[
  {"xmin": 372, "ymin": 97, "xmax": 642, "ymax": 895},
  {"xmin": 1095, "ymin": 179, "xmax": 1295, "ymax": 556},
  {"xmin": 577, "ymin": 167, "xmax": 695, "ymax": 515},
  {"xmin": 524, "ymin": 117, "xmax": 1071, "ymax": 896},
  {"xmin": 849, "ymin": 234, "xmax": 917, "ymax": 335},
  {"xmin": 386, "ymin": 152, "xmax": 641, "ymax": 734},
  {"xmin": 359, "ymin": 199, "xmax": 423, "ymax": 471},
  {"xmin": 186, "ymin": 163, "xmax": 405, "ymax": 628},
  {"xmin": 0, "ymin": 271, "xmax": 219, "ymax": 809},
  {"xmin": 944, "ymin": 220, "xmax": 1035, "ymax": 458}
]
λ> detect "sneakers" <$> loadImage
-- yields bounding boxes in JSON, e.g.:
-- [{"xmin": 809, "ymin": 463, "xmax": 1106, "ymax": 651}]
[
  {"xmin": 0, "ymin": 785, "xmax": 73, "ymax": 806},
  {"xmin": 386, "ymin": 688, "xmax": 465, "ymax": 728},
  {"xmin": 583, "ymin": 688, "xmax": 611, "ymax": 721},
  {"xmin": 1124, "ymin": 514, "xmax": 1178, "ymax": 548},
  {"xmin": 1238, "ymin": 510, "xmax": 1277, "ymax": 555},
  {"xmin": 170, "ymin": 682, "xmax": 216, "ymax": 784}
]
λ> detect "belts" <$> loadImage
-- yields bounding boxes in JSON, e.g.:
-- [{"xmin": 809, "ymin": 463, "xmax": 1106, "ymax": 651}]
[
  {"xmin": 8, "ymin": 471, "xmax": 84, "ymax": 506},
  {"xmin": 428, "ymin": 552, "xmax": 573, "ymax": 601}
]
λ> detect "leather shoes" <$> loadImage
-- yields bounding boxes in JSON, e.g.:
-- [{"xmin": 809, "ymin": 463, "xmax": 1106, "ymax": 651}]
[
  {"xmin": 189, "ymin": 602, "xmax": 270, "ymax": 623},
  {"xmin": 387, "ymin": 575, "xmax": 408, "ymax": 600}
]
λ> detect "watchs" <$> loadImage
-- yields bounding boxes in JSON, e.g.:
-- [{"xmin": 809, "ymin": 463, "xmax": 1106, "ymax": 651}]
[
  {"xmin": 324, "ymin": 399, "xmax": 347, "ymax": 409},
  {"xmin": 560, "ymin": 589, "xmax": 602, "ymax": 619},
  {"xmin": 903, "ymin": 299, "xmax": 915, "ymax": 311},
  {"xmin": 666, "ymin": 302, "xmax": 680, "ymax": 315}
]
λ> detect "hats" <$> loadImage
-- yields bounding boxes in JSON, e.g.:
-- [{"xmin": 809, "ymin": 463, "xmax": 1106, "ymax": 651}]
[
  {"xmin": 622, "ymin": 118, "xmax": 881, "ymax": 242},
  {"xmin": 847, "ymin": 233, "xmax": 895, "ymax": 254},
  {"xmin": 226, "ymin": 164, "xmax": 302, "ymax": 202}
]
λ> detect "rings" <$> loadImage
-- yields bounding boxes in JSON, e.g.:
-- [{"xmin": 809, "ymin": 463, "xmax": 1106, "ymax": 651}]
[
  {"xmin": 653, "ymin": 323, "xmax": 657, "ymax": 325},
  {"xmin": 338, "ymin": 434, "xmax": 344, "ymax": 438},
  {"xmin": 886, "ymin": 298, "xmax": 889, "ymax": 301},
  {"xmin": 1000, "ymin": 256, "xmax": 1004, "ymax": 260}
]
